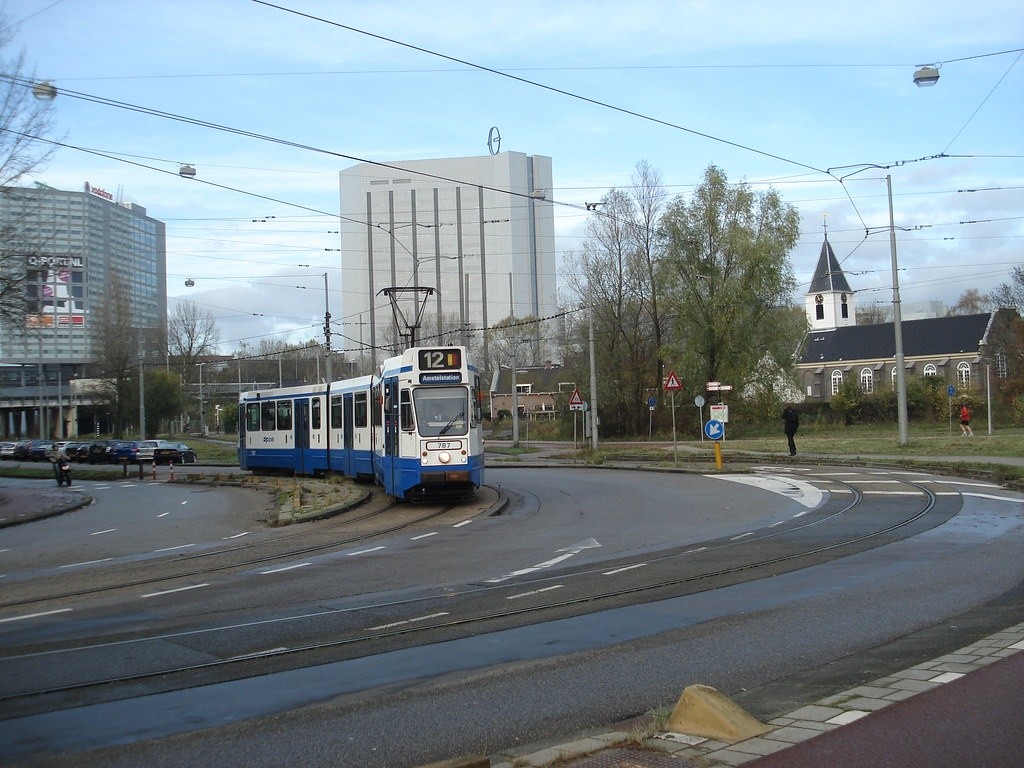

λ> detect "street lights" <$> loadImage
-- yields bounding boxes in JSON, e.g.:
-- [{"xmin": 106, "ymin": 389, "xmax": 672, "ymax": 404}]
[
  {"xmin": 195, "ymin": 362, "xmax": 206, "ymax": 438},
  {"xmin": 235, "ymin": 349, "xmax": 244, "ymax": 397}
]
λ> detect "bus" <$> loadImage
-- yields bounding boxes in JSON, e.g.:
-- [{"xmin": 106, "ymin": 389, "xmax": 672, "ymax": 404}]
[{"xmin": 235, "ymin": 286, "xmax": 488, "ymax": 506}]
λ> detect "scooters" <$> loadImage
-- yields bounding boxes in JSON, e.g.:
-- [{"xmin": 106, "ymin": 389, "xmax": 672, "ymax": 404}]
[{"xmin": 52, "ymin": 457, "xmax": 72, "ymax": 486}]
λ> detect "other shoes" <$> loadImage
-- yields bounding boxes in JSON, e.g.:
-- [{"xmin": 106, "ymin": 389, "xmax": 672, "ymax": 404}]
[
  {"xmin": 789, "ymin": 450, "xmax": 796, "ymax": 456},
  {"xmin": 961, "ymin": 432, "xmax": 967, "ymax": 436},
  {"xmin": 968, "ymin": 433, "xmax": 973, "ymax": 436}
]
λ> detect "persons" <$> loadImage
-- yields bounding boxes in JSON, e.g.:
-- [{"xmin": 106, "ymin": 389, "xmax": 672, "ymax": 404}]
[
  {"xmin": 959, "ymin": 405, "xmax": 973, "ymax": 437},
  {"xmin": 428, "ymin": 404, "xmax": 453, "ymax": 423},
  {"xmin": 782, "ymin": 403, "xmax": 799, "ymax": 456},
  {"xmin": 50, "ymin": 444, "xmax": 71, "ymax": 483}
]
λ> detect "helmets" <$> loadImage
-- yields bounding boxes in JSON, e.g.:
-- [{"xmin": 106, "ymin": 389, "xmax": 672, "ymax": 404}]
[{"xmin": 51, "ymin": 443, "xmax": 59, "ymax": 448}]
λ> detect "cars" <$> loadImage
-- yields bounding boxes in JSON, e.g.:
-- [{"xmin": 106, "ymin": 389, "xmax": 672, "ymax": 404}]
[
  {"xmin": 153, "ymin": 442, "xmax": 197, "ymax": 465},
  {"xmin": 135, "ymin": 438, "xmax": 172, "ymax": 462},
  {"xmin": 112, "ymin": 440, "xmax": 142, "ymax": 464},
  {"xmin": 0, "ymin": 438, "xmax": 120, "ymax": 465}
]
